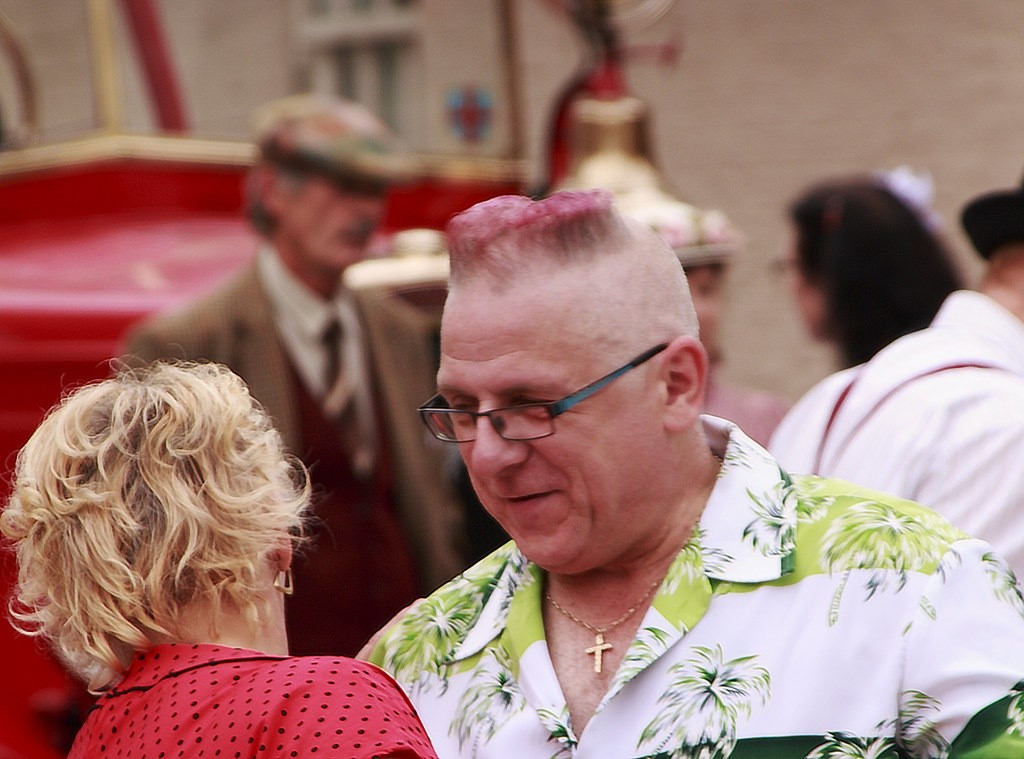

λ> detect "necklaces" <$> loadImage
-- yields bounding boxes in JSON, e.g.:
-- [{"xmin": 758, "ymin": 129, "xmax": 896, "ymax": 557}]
[{"xmin": 542, "ymin": 447, "xmax": 726, "ymax": 675}]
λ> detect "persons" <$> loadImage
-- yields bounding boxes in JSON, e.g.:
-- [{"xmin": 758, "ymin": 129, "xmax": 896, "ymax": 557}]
[
  {"xmin": 772, "ymin": 171, "xmax": 971, "ymax": 373},
  {"xmin": 769, "ymin": 178, "xmax": 1024, "ymax": 590},
  {"xmin": 3, "ymin": 359, "xmax": 438, "ymax": 759},
  {"xmin": 351, "ymin": 190, "xmax": 1024, "ymax": 759},
  {"xmin": 111, "ymin": 91, "xmax": 462, "ymax": 684},
  {"xmin": 678, "ymin": 258, "xmax": 790, "ymax": 451}
]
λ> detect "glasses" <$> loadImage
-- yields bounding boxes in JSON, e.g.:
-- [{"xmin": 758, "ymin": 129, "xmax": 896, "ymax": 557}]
[
  {"xmin": 327, "ymin": 173, "xmax": 388, "ymax": 202},
  {"xmin": 417, "ymin": 343, "xmax": 669, "ymax": 444}
]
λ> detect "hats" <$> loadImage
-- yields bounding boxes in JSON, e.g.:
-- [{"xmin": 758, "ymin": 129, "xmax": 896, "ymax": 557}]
[
  {"xmin": 959, "ymin": 172, "xmax": 1024, "ymax": 262},
  {"xmin": 251, "ymin": 91, "xmax": 425, "ymax": 187}
]
